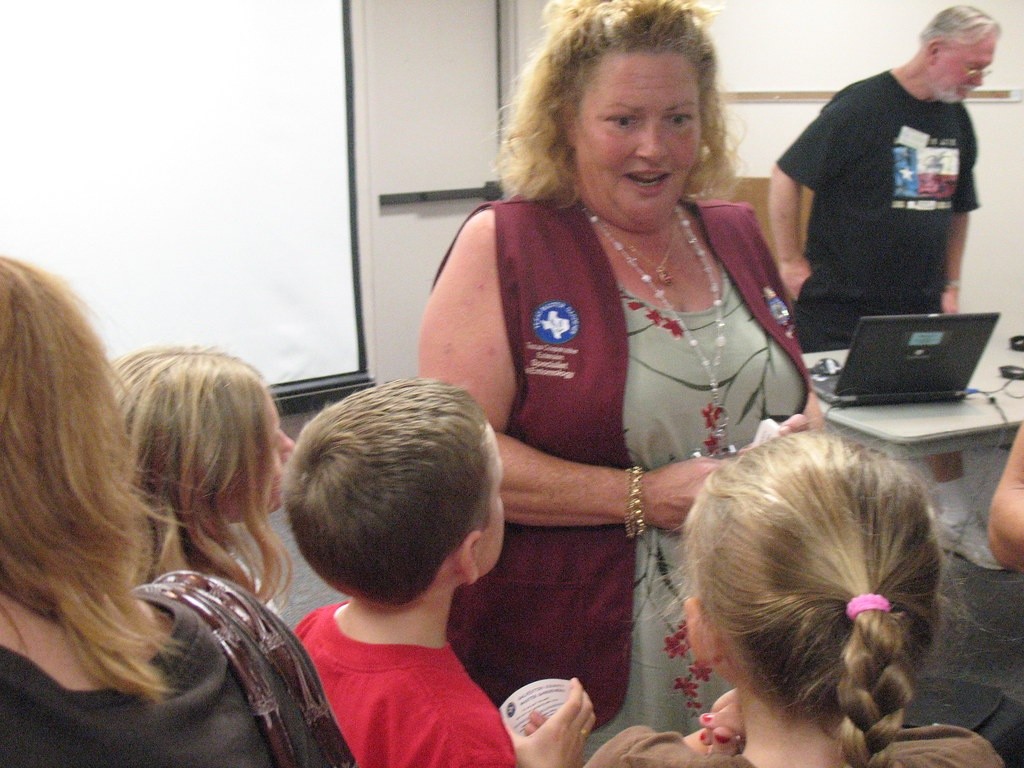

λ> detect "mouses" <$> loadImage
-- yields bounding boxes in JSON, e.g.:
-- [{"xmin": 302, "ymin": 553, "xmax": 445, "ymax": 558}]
[{"xmin": 813, "ymin": 358, "xmax": 842, "ymax": 376}]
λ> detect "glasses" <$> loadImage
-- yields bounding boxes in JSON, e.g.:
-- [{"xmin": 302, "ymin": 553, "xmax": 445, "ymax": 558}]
[{"xmin": 947, "ymin": 40, "xmax": 992, "ymax": 79}]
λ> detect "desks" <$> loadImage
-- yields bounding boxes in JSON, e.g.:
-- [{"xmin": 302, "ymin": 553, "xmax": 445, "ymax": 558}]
[{"xmin": 801, "ymin": 340, "xmax": 1024, "ymax": 570}]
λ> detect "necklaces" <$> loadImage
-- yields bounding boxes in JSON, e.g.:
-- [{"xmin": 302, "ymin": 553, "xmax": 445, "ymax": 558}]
[
  {"xmin": 611, "ymin": 223, "xmax": 678, "ymax": 287},
  {"xmin": 583, "ymin": 203, "xmax": 730, "ymax": 439}
]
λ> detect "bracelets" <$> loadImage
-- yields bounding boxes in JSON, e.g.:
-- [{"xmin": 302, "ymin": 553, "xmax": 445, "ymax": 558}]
[{"xmin": 623, "ymin": 465, "xmax": 647, "ymax": 539}]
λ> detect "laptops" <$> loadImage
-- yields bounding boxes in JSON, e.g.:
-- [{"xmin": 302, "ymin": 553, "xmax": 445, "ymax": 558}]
[{"xmin": 807, "ymin": 311, "xmax": 998, "ymax": 408}]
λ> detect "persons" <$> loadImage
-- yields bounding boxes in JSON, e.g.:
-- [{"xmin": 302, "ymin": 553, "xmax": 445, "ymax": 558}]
[
  {"xmin": 114, "ymin": 342, "xmax": 295, "ymax": 616},
  {"xmin": 768, "ymin": 6, "xmax": 999, "ymax": 354},
  {"xmin": 0, "ymin": 254, "xmax": 353, "ymax": 768},
  {"xmin": 583, "ymin": 428, "xmax": 1007, "ymax": 768},
  {"xmin": 415, "ymin": 0, "xmax": 821, "ymax": 768},
  {"xmin": 987, "ymin": 421, "xmax": 1024, "ymax": 574},
  {"xmin": 280, "ymin": 376, "xmax": 598, "ymax": 768}
]
